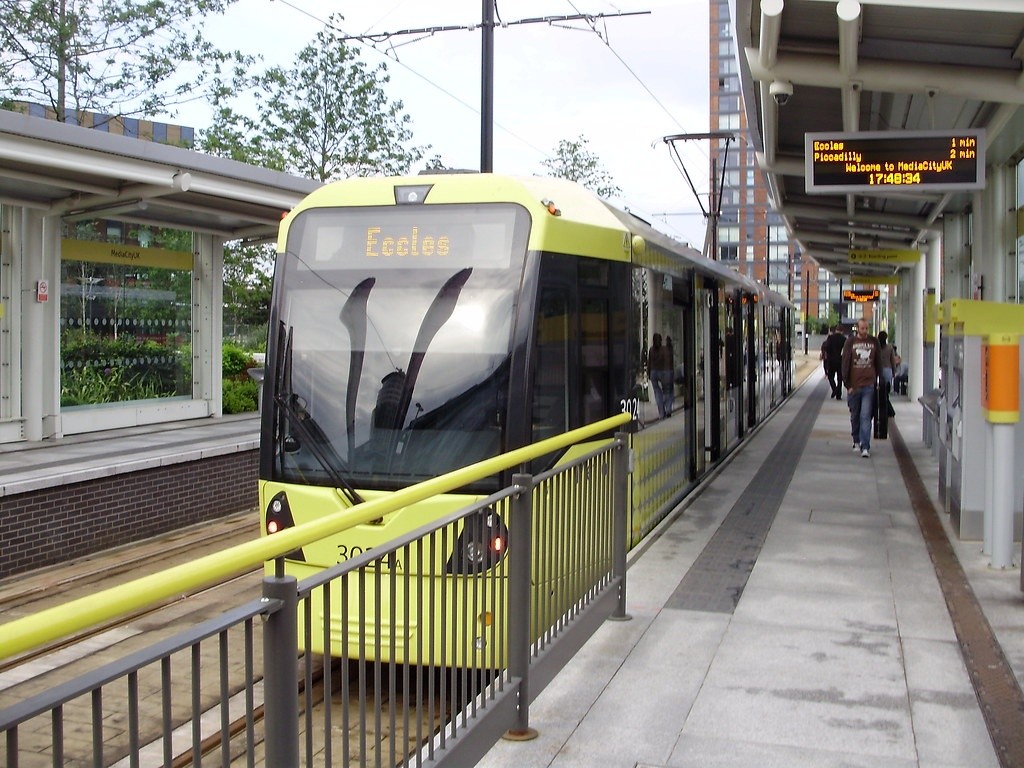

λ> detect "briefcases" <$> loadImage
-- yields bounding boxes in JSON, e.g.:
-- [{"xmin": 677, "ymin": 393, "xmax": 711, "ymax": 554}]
[{"xmin": 874, "ymin": 387, "xmax": 888, "ymax": 439}]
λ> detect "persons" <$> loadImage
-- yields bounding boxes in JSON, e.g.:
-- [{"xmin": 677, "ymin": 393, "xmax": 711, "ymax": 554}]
[
  {"xmin": 699, "ymin": 325, "xmax": 763, "ymax": 402},
  {"xmin": 840, "ymin": 317, "xmax": 884, "ymax": 457},
  {"xmin": 878, "ymin": 330, "xmax": 897, "ymax": 390},
  {"xmin": 776, "ymin": 334, "xmax": 782, "ymax": 367},
  {"xmin": 822, "ymin": 324, "xmax": 847, "ymax": 401},
  {"xmin": 646, "ymin": 332, "xmax": 673, "ymax": 419},
  {"xmin": 890, "ymin": 345, "xmax": 908, "ymax": 394}
]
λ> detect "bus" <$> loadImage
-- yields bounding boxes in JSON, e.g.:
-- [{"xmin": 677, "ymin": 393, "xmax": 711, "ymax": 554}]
[{"xmin": 258, "ymin": 172, "xmax": 797, "ymax": 671}]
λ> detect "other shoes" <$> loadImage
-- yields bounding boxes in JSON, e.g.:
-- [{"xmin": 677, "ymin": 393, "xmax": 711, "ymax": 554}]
[
  {"xmin": 861, "ymin": 447, "xmax": 871, "ymax": 457},
  {"xmin": 853, "ymin": 441, "xmax": 861, "ymax": 451},
  {"xmin": 830, "ymin": 388, "xmax": 841, "ymax": 399}
]
